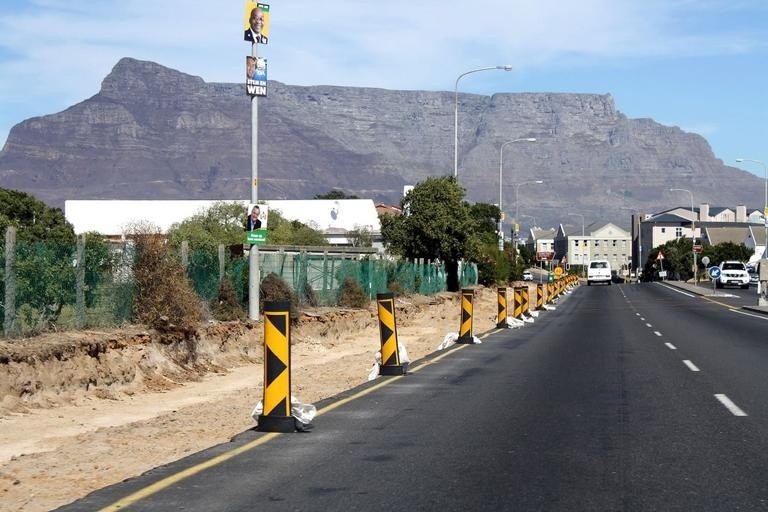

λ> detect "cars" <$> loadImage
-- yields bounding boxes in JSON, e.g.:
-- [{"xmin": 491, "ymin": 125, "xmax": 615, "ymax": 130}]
[
  {"xmin": 522, "ymin": 270, "xmax": 534, "ymax": 281},
  {"xmin": 612, "ymin": 274, "xmax": 640, "ymax": 284}
]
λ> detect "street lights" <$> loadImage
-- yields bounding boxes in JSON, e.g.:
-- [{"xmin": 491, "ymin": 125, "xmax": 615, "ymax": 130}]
[
  {"xmin": 735, "ymin": 157, "xmax": 768, "ymax": 259},
  {"xmin": 567, "ymin": 212, "xmax": 587, "ymax": 279},
  {"xmin": 497, "ymin": 137, "xmax": 537, "ymax": 248},
  {"xmin": 669, "ymin": 187, "xmax": 700, "ymax": 287},
  {"xmin": 513, "ymin": 180, "xmax": 545, "ymax": 250},
  {"xmin": 619, "ymin": 206, "xmax": 642, "ymax": 271},
  {"xmin": 453, "ymin": 63, "xmax": 513, "ymax": 181}
]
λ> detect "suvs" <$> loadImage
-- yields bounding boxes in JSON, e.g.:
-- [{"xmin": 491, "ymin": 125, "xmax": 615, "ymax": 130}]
[
  {"xmin": 718, "ymin": 260, "xmax": 751, "ymax": 289},
  {"xmin": 745, "ymin": 265, "xmax": 755, "ymax": 272},
  {"xmin": 748, "ymin": 272, "xmax": 760, "ymax": 286}
]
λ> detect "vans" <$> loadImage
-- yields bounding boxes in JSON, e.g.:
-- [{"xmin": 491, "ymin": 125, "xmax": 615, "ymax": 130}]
[{"xmin": 585, "ymin": 261, "xmax": 613, "ymax": 286}]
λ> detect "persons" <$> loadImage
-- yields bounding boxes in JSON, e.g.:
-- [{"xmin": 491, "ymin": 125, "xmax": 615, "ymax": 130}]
[
  {"xmin": 246, "ymin": 55, "xmax": 265, "ymax": 80},
  {"xmin": 247, "ymin": 205, "xmax": 261, "ymax": 231},
  {"xmin": 244, "ymin": 7, "xmax": 268, "ymax": 44}
]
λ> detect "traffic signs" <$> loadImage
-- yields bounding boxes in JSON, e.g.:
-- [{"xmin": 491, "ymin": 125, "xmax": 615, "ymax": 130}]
[
  {"xmin": 535, "ymin": 251, "xmax": 557, "ymax": 262},
  {"xmin": 692, "ymin": 244, "xmax": 704, "ymax": 254}
]
